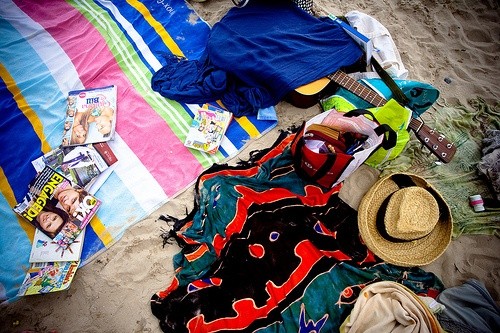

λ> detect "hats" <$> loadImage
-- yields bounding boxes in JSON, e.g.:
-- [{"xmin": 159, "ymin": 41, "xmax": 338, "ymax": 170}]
[{"xmin": 358, "ymin": 171, "xmax": 454, "ymax": 268}]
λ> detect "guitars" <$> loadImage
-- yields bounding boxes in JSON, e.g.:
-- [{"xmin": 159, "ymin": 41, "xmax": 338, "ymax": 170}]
[{"xmin": 288, "ymin": 70, "xmax": 456, "ymax": 164}]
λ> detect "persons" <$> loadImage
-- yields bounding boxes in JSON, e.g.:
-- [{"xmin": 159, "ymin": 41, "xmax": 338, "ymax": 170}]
[
  {"xmin": 70, "ymin": 110, "xmax": 94, "ymax": 144},
  {"xmin": 21, "ymin": 194, "xmax": 70, "ymax": 240},
  {"xmin": 43, "ymin": 173, "xmax": 88, "ymax": 218},
  {"xmin": 94, "ymin": 106, "xmax": 114, "ymax": 135}
]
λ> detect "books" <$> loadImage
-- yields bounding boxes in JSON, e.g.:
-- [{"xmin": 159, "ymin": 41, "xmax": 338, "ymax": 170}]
[
  {"xmin": 12, "ymin": 83, "xmax": 117, "ymax": 297},
  {"xmin": 183, "ymin": 104, "xmax": 233, "ymax": 155}
]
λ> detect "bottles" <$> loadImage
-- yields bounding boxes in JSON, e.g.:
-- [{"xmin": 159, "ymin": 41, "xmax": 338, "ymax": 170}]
[{"xmin": 470, "ymin": 195, "xmax": 485, "ymax": 211}]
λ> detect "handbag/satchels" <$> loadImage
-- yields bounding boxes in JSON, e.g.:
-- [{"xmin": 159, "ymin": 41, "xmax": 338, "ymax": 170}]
[{"xmin": 288, "ymin": 107, "xmax": 397, "ymax": 191}]
